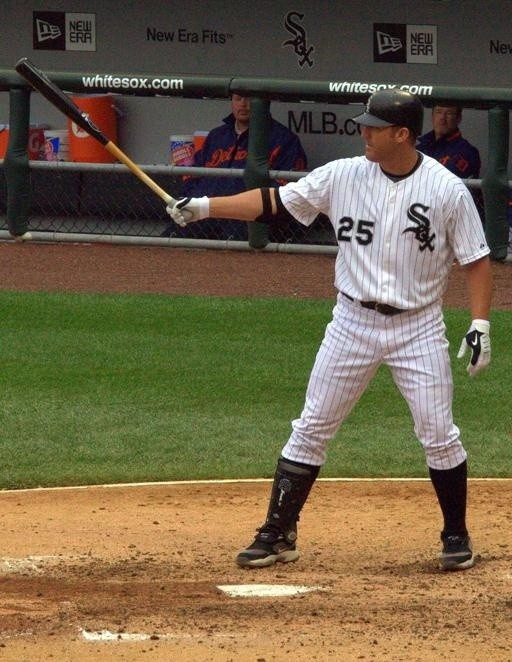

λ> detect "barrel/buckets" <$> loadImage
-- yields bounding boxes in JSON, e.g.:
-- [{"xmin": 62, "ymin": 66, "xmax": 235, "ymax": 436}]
[
  {"xmin": 0, "ymin": 124, "xmax": 45, "ymax": 158},
  {"xmin": 170, "ymin": 134, "xmax": 195, "ymax": 167},
  {"xmin": 44, "ymin": 130, "xmax": 68, "ymax": 162},
  {"xmin": 193, "ymin": 130, "xmax": 210, "ymax": 155},
  {"xmin": 68, "ymin": 94, "xmax": 122, "ymax": 163}
]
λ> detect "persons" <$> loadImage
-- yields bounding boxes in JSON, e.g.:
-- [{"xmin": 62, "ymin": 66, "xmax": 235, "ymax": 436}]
[
  {"xmin": 160, "ymin": 93, "xmax": 309, "ymax": 238},
  {"xmin": 414, "ymin": 102, "xmax": 487, "ymax": 223},
  {"xmin": 166, "ymin": 91, "xmax": 493, "ymax": 571}
]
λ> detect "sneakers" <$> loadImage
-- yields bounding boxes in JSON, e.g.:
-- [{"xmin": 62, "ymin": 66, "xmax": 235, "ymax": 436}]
[
  {"xmin": 437, "ymin": 532, "xmax": 475, "ymax": 571},
  {"xmin": 236, "ymin": 529, "xmax": 300, "ymax": 569}
]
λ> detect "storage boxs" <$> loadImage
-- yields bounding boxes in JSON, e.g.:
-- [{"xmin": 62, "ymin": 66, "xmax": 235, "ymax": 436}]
[{"xmin": 0, "ymin": 123, "xmax": 49, "ymax": 160}]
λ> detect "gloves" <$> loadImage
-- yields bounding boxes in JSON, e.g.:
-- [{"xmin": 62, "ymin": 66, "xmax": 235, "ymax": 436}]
[
  {"xmin": 456, "ymin": 319, "xmax": 492, "ymax": 377},
  {"xmin": 166, "ymin": 195, "xmax": 210, "ymax": 227}
]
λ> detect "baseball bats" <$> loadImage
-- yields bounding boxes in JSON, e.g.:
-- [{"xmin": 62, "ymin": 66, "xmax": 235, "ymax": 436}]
[{"xmin": 14, "ymin": 57, "xmax": 179, "ymax": 208}]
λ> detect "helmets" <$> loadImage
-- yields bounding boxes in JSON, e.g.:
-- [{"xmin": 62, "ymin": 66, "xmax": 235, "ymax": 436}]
[{"xmin": 353, "ymin": 87, "xmax": 423, "ymax": 136}]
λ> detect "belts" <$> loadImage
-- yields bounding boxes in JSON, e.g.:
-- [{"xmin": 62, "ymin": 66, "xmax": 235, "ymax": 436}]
[{"xmin": 341, "ymin": 290, "xmax": 408, "ymax": 316}]
